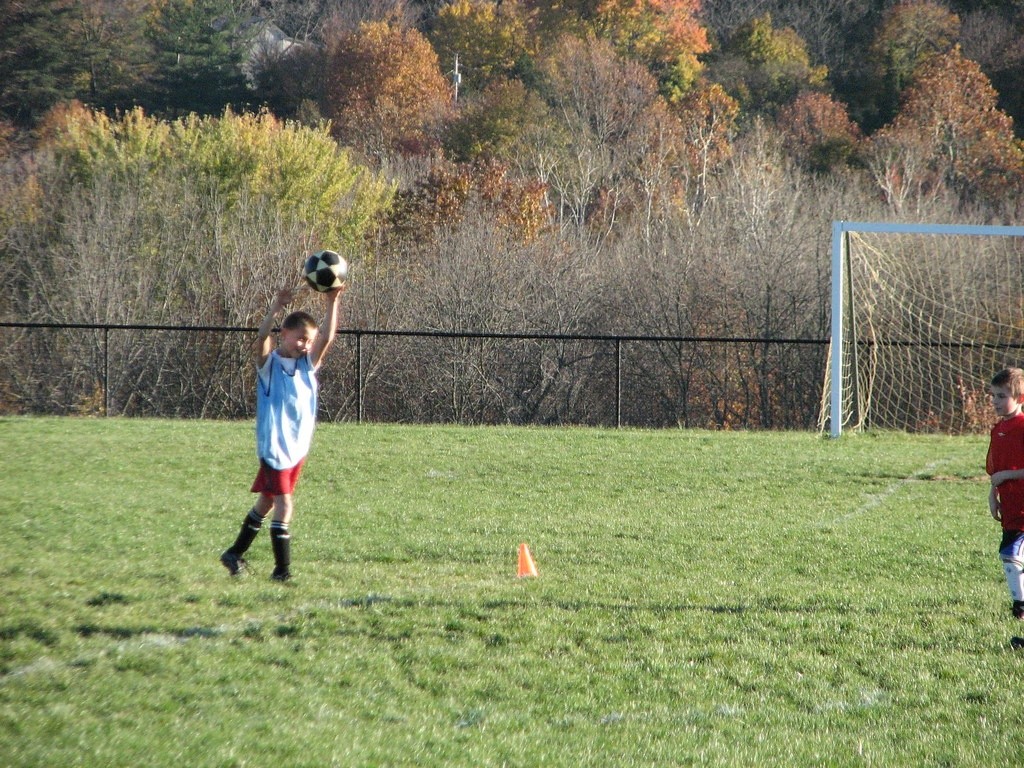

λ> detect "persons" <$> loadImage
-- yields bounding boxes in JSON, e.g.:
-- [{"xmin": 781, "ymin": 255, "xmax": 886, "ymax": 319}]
[
  {"xmin": 983, "ymin": 366, "xmax": 1024, "ymax": 652},
  {"xmin": 219, "ymin": 272, "xmax": 348, "ymax": 597}
]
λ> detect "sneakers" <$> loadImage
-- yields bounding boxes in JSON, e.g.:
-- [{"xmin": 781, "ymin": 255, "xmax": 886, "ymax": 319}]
[
  {"xmin": 220, "ymin": 550, "xmax": 245, "ymax": 576},
  {"xmin": 269, "ymin": 570, "xmax": 291, "ymax": 583}
]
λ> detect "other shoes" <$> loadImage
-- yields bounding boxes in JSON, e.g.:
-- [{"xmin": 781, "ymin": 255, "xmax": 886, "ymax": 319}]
[{"xmin": 1011, "ymin": 637, "xmax": 1024, "ymax": 650}]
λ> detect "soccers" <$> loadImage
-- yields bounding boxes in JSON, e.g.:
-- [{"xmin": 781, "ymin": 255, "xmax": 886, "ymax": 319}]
[{"xmin": 304, "ymin": 250, "xmax": 348, "ymax": 293}]
